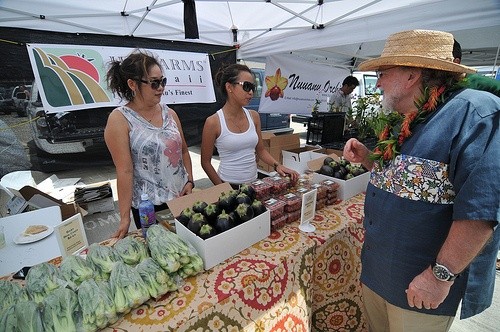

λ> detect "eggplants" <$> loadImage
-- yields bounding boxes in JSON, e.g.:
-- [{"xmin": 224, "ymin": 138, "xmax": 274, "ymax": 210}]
[
  {"xmin": 317, "ymin": 157, "xmax": 368, "ymax": 181},
  {"xmin": 178, "ymin": 184, "xmax": 266, "ymax": 240}
]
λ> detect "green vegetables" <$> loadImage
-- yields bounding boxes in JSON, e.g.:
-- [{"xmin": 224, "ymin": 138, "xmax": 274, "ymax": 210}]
[{"xmin": 0, "ymin": 224, "xmax": 203, "ymax": 332}]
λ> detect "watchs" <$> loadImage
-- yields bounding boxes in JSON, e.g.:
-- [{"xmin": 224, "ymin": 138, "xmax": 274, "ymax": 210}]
[{"xmin": 431, "ymin": 257, "xmax": 460, "ymax": 282}]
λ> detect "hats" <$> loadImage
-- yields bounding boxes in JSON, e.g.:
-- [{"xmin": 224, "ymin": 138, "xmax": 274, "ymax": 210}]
[{"xmin": 357, "ymin": 29, "xmax": 477, "ymax": 74}]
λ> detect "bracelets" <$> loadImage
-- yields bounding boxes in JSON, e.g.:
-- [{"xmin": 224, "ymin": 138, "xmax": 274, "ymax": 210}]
[
  {"xmin": 186, "ymin": 181, "xmax": 195, "ymax": 189},
  {"xmin": 274, "ymin": 162, "xmax": 281, "ymax": 172}
]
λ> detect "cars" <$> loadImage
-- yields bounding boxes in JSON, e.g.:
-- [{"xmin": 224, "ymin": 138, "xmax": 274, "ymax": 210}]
[{"xmin": 0, "ymin": 79, "xmax": 111, "ymax": 173}]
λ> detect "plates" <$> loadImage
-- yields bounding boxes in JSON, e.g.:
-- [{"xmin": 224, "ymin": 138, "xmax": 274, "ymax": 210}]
[{"xmin": 13, "ymin": 225, "xmax": 53, "ymax": 245}]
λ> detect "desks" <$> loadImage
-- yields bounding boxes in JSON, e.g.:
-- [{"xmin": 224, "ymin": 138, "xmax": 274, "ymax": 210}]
[
  {"xmin": 0, "ymin": 191, "xmax": 369, "ymax": 332},
  {"xmin": 0, "ymin": 203, "xmax": 65, "ymax": 281}
]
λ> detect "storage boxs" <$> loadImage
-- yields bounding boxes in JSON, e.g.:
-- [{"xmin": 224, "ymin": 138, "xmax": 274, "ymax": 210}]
[
  {"xmin": 0, "ymin": 185, "xmax": 65, "ymax": 218},
  {"xmin": 75, "ymin": 176, "xmax": 113, "ymax": 217},
  {"xmin": 304, "ymin": 156, "xmax": 374, "ymax": 201},
  {"xmin": 257, "ymin": 128, "xmax": 301, "ymax": 173},
  {"xmin": 281, "ymin": 145, "xmax": 325, "ymax": 175},
  {"xmin": 312, "ymin": 147, "xmax": 343, "ymax": 161},
  {"xmin": 163, "ymin": 180, "xmax": 274, "ymax": 272}
]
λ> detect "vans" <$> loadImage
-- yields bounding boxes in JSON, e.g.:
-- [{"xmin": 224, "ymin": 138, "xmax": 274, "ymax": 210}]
[{"xmin": 351, "ymin": 71, "xmax": 384, "ymax": 117}]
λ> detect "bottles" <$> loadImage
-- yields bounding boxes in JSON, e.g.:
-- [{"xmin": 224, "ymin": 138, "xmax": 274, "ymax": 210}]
[{"xmin": 139, "ymin": 194, "xmax": 157, "ymax": 236}]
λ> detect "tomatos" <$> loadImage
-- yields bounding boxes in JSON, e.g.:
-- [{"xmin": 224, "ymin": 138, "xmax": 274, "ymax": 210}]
[{"xmin": 251, "ymin": 177, "xmax": 339, "ymax": 231}]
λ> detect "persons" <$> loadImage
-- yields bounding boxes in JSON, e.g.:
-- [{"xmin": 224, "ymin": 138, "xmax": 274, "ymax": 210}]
[
  {"xmin": 342, "ymin": 29, "xmax": 500, "ymax": 332},
  {"xmin": 201, "ymin": 63, "xmax": 299, "ymax": 190},
  {"xmin": 453, "ymin": 39, "xmax": 477, "ymax": 79},
  {"xmin": 19, "ymin": 85, "xmax": 31, "ymax": 99},
  {"xmin": 328, "ymin": 76, "xmax": 359, "ymax": 124},
  {"xmin": 104, "ymin": 49, "xmax": 195, "ymax": 239}
]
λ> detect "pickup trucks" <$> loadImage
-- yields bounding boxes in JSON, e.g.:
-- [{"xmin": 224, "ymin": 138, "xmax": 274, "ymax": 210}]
[{"xmin": 242, "ymin": 67, "xmax": 291, "ymax": 131}]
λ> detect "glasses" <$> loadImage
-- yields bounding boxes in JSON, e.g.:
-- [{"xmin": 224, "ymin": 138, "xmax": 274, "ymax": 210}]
[
  {"xmin": 232, "ymin": 81, "xmax": 256, "ymax": 93},
  {"xmin": 130, "ymin": 77, "xmax": 167, "ymax": 89},
  {"xmin": 376, "ymin": 66, "xmax": 400, "ymax": 79}
]
